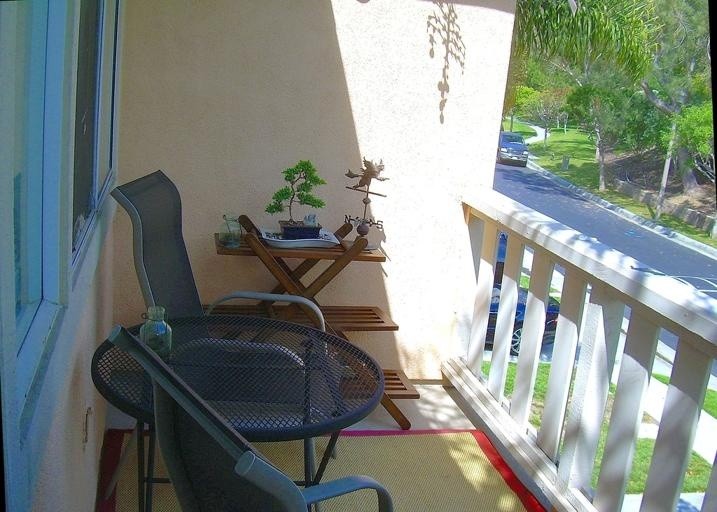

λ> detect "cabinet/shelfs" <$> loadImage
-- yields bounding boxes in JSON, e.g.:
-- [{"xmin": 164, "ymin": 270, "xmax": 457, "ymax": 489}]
[{"xmin": 202, "ymin": 215, "xmax": 420, "ymax": 430}]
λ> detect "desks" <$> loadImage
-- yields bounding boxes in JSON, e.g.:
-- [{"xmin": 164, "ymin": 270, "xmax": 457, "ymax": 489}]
[{"xmin": 90, "ymin": 304, "xmax": 384, "ymax": 512}]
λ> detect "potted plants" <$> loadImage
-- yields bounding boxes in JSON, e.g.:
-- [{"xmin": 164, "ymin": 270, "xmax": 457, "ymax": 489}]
[{"xmin": 266, "ymin": 162, "xmax": 329, "ymax": 240}]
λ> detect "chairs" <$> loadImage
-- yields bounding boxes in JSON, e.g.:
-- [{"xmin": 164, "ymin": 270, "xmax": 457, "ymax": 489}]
[
  {"xmin": 105, "ymin": 324, "xmax": 395, "ymax": 512},
  {"xmin": 108, "ymin": 167, "xmax": 349, "ymax": 459}
]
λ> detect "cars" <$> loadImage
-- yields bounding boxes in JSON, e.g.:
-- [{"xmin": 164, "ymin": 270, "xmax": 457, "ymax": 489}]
[{"xmin": 484, "ymin": 288, "xmax": 560, "ymax": 356}]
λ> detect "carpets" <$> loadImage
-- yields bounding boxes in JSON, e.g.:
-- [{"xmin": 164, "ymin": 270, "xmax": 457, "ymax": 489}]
[{"xmin": 94, "ymin": 428, "xmax": 542, "ymax": 512}]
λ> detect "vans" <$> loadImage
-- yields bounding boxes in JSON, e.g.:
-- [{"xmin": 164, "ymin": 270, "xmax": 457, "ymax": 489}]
[{"xmin": 496, "ymin": 133, "xmax": 530, "ymax": 167}]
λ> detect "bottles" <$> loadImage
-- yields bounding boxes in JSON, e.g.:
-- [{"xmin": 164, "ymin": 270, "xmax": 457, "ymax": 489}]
[
  {"xmin": 140, "ymin": 305, "xmax": 173, "ymax": 365},
  {"xmin": 219, "ymin": 219, "xmax": 241, "ymax": 248}
]
